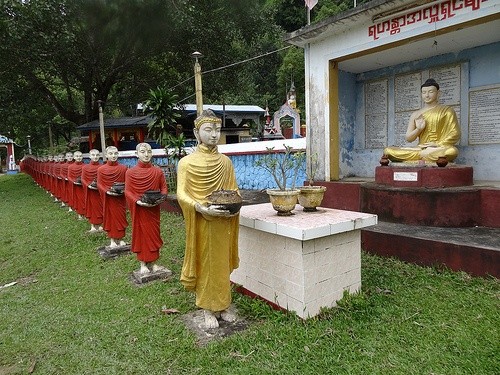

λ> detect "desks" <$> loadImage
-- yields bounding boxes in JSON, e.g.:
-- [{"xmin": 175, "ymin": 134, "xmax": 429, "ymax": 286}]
[{"xmin": 230, "ymin": 202, "xmax": 378, "ymax": 321}]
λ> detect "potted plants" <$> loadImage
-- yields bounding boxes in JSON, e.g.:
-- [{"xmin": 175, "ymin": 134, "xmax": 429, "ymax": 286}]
[
  {"xmin": 291, "ymin": 151, "xmax": 327, "ymax": 212},
  {"xmin": 254, "ymin": 143, "xmax": 307, "ymax": 216}
]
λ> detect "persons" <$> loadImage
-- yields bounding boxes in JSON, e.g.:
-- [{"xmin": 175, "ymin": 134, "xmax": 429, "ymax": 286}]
[
  {"xmin": 68, "ymin": 150, "xmax": 87, "ymax": 220},
  {"xmin": 97, "ymin": 146, "xmax": 128, "ymax": 247},
  {"xmin": 59, "ymin": 151, "xmax": 75, "ymax": 211},
  {"xmin": 20, "ymin": 149, "xmax": 60, "ymax": 201},
  {"xmin": 383, "ymin": 78, "xmax": 462, "ymax": 168},
  {"xmin": 124, "ymin": 141, "xmax": 168, "ymax": 274},
  {"xmin": 53, "ymin": 153, "xmax": 67, "ymax": 207},
  {"xmin": 81, "ymin": 149, "xmax": 106, "ymax": 233},
  {"xmin": 175, "ymin": 108, "xmax": 245, "ymax": 331}
]
